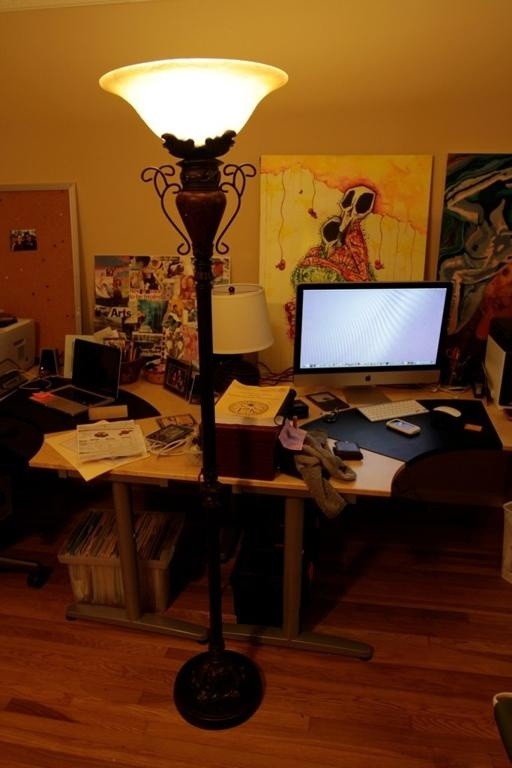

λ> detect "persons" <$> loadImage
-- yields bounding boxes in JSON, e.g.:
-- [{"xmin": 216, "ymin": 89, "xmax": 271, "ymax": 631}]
[{"xmin": 12, "ymin": 233, "xmax": 37, "ymax": 250}]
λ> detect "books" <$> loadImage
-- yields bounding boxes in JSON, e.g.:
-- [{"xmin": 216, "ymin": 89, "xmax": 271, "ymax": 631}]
[
  {"xmin": 214, "ymin": 378, "xmax": 296, "ymax": 427},
  {"xmin": 44, "ymin": 420, "xmax": 195, "ymax": 482},
  {"xmin": 58, "ymin": 511, "xmax": 181, "ymax": 610}
]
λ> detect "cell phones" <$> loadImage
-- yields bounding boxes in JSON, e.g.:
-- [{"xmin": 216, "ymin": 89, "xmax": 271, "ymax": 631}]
[{"xmin": 386, "ymin": 418, "xmax": 421, "ymax": 435}]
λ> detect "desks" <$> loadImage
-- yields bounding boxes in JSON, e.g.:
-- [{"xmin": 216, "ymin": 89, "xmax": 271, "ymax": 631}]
[{"xmin": 29, "ymin": 371, "xmax": 512, "ymax": 661}]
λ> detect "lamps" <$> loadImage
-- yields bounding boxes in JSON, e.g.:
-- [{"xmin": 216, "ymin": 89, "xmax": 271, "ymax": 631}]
[
  {"xmin": 96, "ymin": 55, "xmax": 293, "ymax": 733},
  {"xmin": 208, "ymin": 281, "xmax": 276, "ymax": 386}
]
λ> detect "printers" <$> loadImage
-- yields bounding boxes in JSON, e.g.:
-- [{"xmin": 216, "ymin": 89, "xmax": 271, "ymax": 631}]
[{"xmin": 0, "ymin": 317, "xmax": 36, "ymax": 372}]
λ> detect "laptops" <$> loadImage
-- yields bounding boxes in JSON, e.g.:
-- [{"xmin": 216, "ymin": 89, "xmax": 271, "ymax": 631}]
[{"xmin": 28, "ymin": 338, "xmax": 122, "ymax": 417}]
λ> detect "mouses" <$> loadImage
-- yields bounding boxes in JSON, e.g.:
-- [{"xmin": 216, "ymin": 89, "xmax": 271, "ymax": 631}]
[{"xmin": 432, "ymin": 404, "xmax": 462, "ymax": 417}]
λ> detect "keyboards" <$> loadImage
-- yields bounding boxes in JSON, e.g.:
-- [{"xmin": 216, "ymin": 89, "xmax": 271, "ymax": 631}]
[{"xmin": 357, "ymin": 399, "xmax": 430, "ymax": 423}]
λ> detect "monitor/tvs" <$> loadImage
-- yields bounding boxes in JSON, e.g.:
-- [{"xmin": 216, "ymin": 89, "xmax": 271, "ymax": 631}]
[{"xmin": 293, "ymin": 282, "xmax": 454, "ymax": 405}]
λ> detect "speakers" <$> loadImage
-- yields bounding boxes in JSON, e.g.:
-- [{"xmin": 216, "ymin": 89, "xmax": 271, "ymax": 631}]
[{"xmin": 38, "ymin": 348, "xmax": 58, "ymax": 376}]
